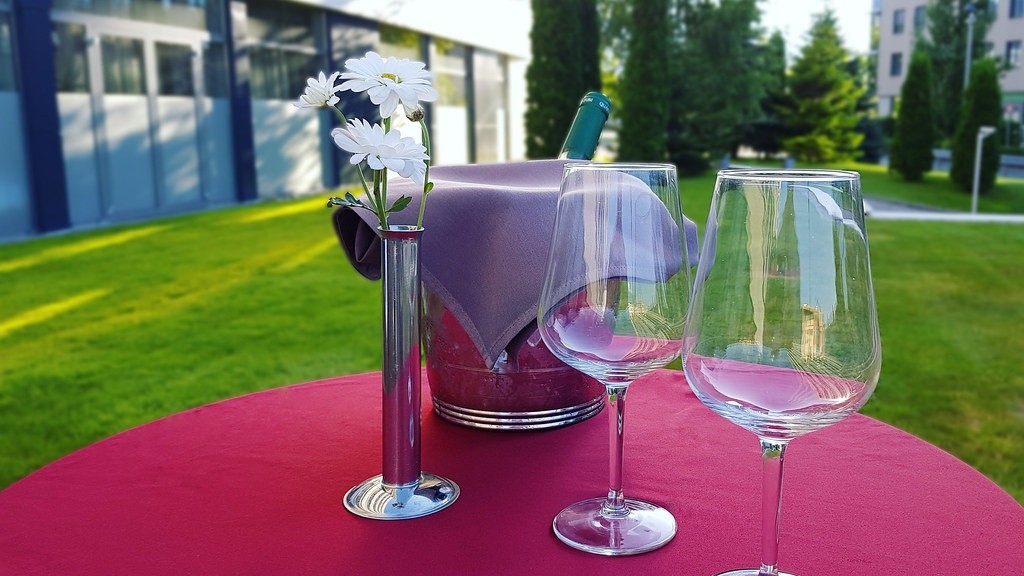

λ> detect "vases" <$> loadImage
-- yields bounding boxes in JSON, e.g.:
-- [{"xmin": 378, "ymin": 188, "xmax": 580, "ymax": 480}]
[{"xmin": 343, "ymin": 228, "xmax": 459, "ymax": 520}]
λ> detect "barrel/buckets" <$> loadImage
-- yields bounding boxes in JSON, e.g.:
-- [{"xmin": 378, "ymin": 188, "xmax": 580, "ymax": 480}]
[{"xmin": 419, "ymin": 276, "xmax": 621, "ymax": 431}]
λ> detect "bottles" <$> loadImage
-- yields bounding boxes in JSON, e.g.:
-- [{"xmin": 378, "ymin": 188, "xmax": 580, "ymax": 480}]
[{"xmin": 557, "ymin": 91, "xmax": 611, "ymax": 161}]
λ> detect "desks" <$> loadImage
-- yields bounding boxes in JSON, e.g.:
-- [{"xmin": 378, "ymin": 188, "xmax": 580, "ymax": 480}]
[{"xmin": 0, "ymin": 367, "xmax": 1024, "ymax": 576}]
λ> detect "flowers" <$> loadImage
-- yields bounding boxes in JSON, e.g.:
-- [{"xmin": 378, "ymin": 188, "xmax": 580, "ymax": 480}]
[{"xmin": 295, "ymin": 50, "xmax": 437, "ymax": 228}]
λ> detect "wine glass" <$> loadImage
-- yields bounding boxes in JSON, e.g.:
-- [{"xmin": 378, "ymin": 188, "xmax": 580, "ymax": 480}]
[
  {"xmin": 682, "ymin": 170, "xmax": 881, "ymax": 576},
  {"xmin": 538, "ymin": 161, "xmax": 693, "ymax": 556}
]
